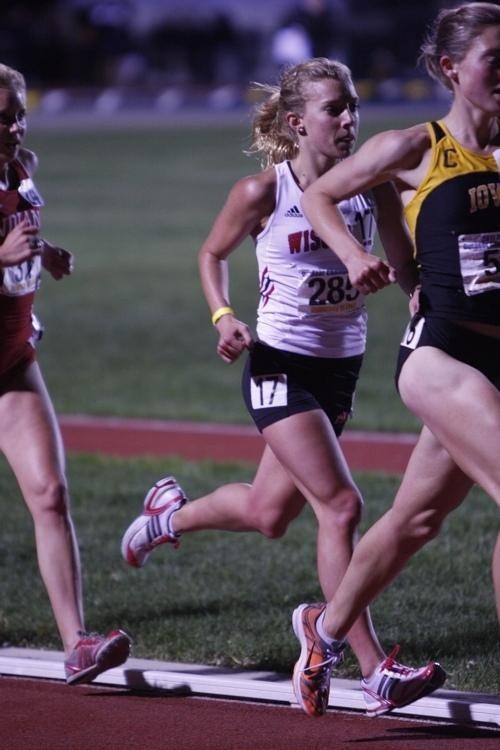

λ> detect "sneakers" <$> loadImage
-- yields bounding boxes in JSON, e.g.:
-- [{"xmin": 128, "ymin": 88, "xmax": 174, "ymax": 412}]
[
  {"xmin": 122, "ymin": 476, "xmax": 187, "ymax": 568},
  {"xmin": 292, "ymin": 603, "xmax": 346, "ymax": 718},
  {"xmin": 361, "ymin": 658, "xmax": 446, "ymax": 717},
  {"xmin": 64, "ymin": 629, "xmax": 130, "ymax": 685}
]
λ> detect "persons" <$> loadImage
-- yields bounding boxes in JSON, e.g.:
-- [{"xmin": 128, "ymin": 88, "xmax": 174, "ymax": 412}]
[
  {"xmin": 120, "ymin": 57, "xmax": 446, "ymax": 718},
  {"xmin": 0, "ymin": 64, "xmax": 131, "ymax": 686},
  {"xmin": 294, "ymin": 2, "xmax": 500, "ymax": 721}
]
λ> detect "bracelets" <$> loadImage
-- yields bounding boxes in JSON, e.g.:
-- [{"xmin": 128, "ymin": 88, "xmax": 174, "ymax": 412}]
[{"xmin": 211, "ymin": 306, "xmax": 235, "ymax": 326}]
